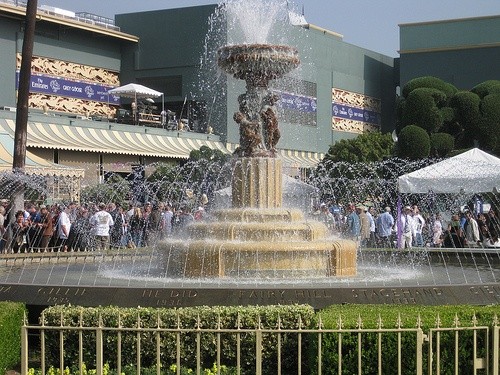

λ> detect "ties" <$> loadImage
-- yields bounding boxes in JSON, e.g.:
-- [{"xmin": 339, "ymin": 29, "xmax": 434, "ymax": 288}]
[{"xmin": 405, "ymin": 215, "xmax": 408, "ymax": 233}]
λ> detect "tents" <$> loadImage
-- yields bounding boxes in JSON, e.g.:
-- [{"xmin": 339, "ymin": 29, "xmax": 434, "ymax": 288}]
[
  {"xmin": 395, "ymin": 148, "xmax": 500, "ymax": 249},
  {"xmin": 214, "ymin": 173, "xmax": 320, "ymax": 217},
  {"xmin": 108, "ymin": 83, "xmax": 164, "ymax": 123}
]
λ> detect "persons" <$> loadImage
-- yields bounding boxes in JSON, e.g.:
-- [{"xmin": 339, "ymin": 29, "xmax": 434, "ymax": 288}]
[
  {"xmin": 319, "ymin": 202, "xmax": 500, "ymax": 248},
  {"xmin": 261, "ymin": 92, "xmax": 281, "ymax": 152},
  {"xmin": 233, "ymin": 112, "xmax": 262, "ymax": 151},
  {"xmin": 0, "ymin": 198, "xmax": 205, "ymax": 251}
]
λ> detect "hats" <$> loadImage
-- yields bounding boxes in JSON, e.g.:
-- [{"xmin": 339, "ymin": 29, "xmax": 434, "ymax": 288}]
[
  {"xmin": 404, "ymin": 206, "xmax": 411, "ymax": 211},
  {"xmin": 39, "ymin": 205, "xmax": 46, "ymax": 209},
  {"xmin": 99, "ymin": 203, "xmax": 105, "ymax": 207},
  {"xmin": 356, "ymin": 206, "xmax": 362, "ymax": 210},
  {"xmin": 384, "ymin": 207, "xmax": 391, "ymax": 211}
]
